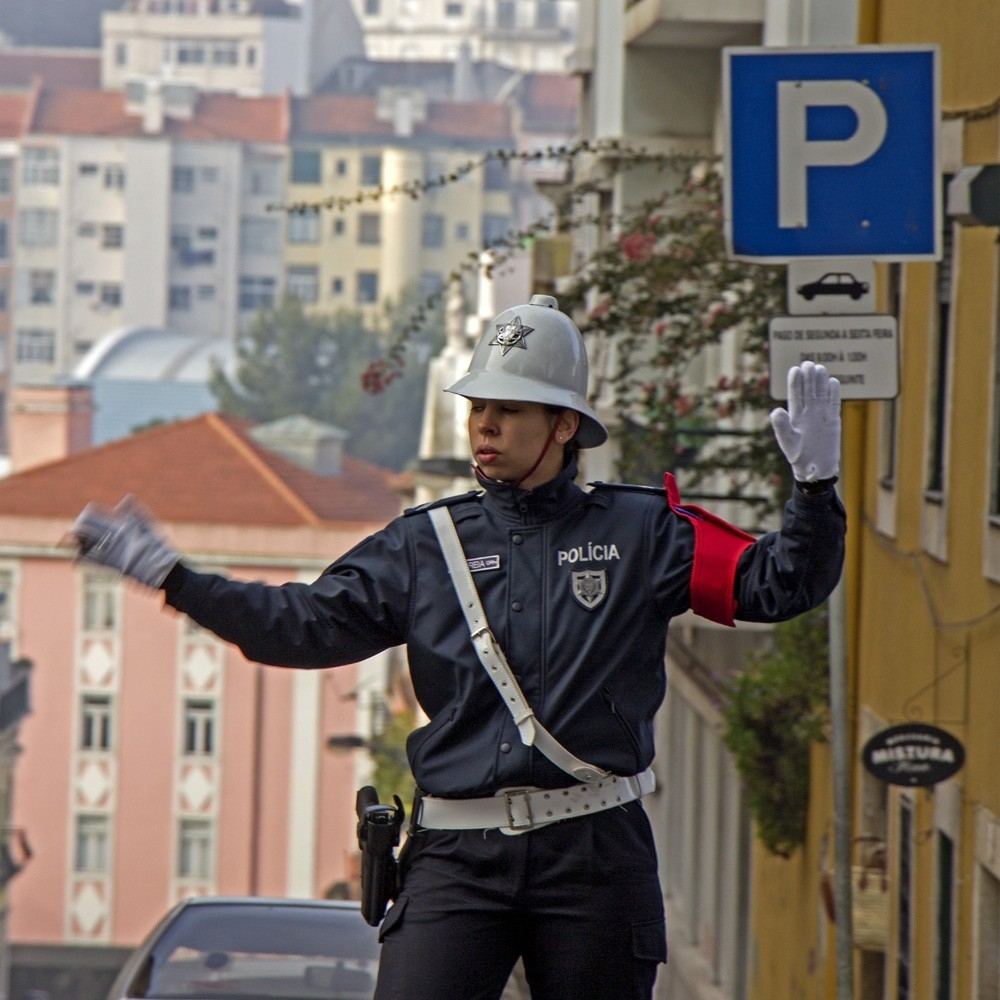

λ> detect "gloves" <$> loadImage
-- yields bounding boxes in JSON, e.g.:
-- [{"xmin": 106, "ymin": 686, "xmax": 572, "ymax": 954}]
[
  {"xmin": 71, "ymin": 498, "xmax": 181, "ymax": 589},
  {"xmin": 770, "ymin": 361, "xmax": 842, "ymax": 481}
]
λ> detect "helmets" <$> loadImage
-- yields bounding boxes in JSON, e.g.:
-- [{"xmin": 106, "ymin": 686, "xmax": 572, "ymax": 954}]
[{"xmin": 443, "ymin": 294, "xmax": 608, "ymax": 449}]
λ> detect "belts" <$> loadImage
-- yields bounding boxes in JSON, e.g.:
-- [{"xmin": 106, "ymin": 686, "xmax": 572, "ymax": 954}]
[{"xmin": 417, "ymin": 776, "xmax": 639, "ymax": 836}]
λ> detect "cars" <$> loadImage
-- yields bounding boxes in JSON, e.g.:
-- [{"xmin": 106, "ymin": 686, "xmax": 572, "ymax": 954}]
[{"xmin": 105, "ymin": 895, "xmax": 391, "ymax": 1000}]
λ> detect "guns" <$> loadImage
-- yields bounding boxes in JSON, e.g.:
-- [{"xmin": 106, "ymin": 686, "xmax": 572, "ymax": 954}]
[{"xmin": 354, "ymin": 784, "xmax": 406, "ymax": 927}]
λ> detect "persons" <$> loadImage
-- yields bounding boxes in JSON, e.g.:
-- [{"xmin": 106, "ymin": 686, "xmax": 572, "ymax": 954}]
[{"xmin": 72, "ymin": 294, "xmax": 848, "ymax": 1000}]
